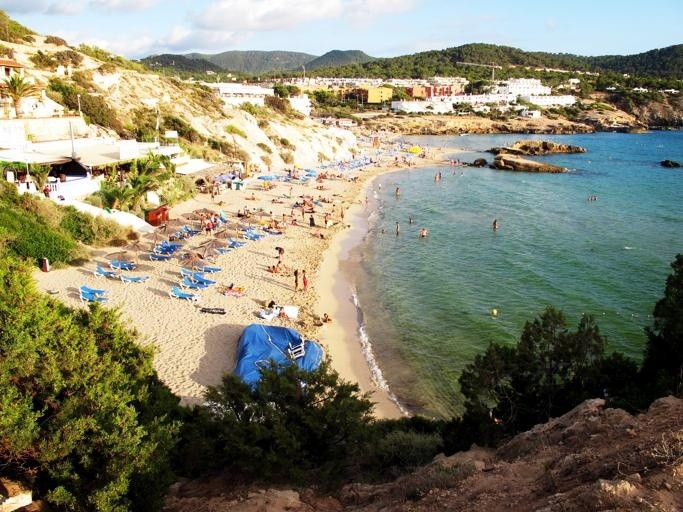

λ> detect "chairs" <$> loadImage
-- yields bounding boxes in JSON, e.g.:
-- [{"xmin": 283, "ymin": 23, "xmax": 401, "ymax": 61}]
[
  {"xmin": 257, "ymin": 155, "xmax": 373, "ymax": 184},
  {"xmin": 77, "ymin": 209, "xmax": 277, "ymax": 303},
  {"xmin": 312, "ymin": 201, "xmax": 321, "ymax": 205}
]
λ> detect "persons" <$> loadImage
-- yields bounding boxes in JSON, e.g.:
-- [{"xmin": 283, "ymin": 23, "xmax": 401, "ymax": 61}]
[
  {"xmin": 395, "ymin": 188, "xmax": 399, "ymax": 196},
  {"xmin": 420, "ymin": 228, "xmax": 428, "ymax": 238},
  {"xmin": 57, "ymin": 194, "xmax": 65, "ymax": 201},
  {"xmin": 493, "ymin": 220, "xmax": 498, "ymax": 230},
  {"xmin": 492, "ymin": 307, "xmax": 497, "ymax": 317},
  {"xmin": 43, "ymin": 185, "xmax": 52, "ymax": 198},
  {"xmin": 393, "ymin": 158, "xmax": 461, "ymax": 181},
  {"xmin": 202, "ymin": 158, "xmax": 379, "ymax": 327},
  {"xmin": 395, "ymin": 222, "xmax": 400, "ymax": 235}
]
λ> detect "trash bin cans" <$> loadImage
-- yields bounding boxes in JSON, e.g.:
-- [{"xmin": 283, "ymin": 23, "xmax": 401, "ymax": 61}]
[
  {"xmin": 158, "ymin": 205, "xmax": 169, "ymax": 221},
  {"xmin": 147, "ymin": 209, "xmax": 161, "ymax": 227}
]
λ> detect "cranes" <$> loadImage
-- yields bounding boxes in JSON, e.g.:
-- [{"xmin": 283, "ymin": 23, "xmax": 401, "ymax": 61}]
[{"xmin": 456, "ymin": 61, "xmax": 502, "ymax": 80}]
